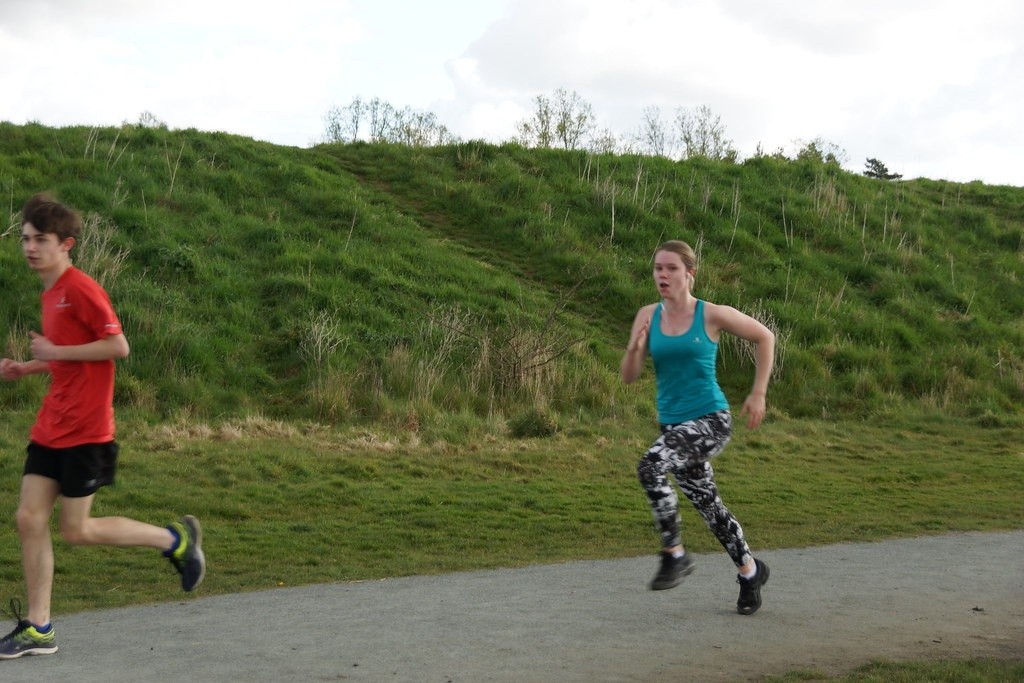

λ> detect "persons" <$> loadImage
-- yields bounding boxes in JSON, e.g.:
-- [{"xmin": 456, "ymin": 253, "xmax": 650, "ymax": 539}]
[
  {"xmin": 621, "ymin": 241, "xmax": 775, "ymax": 615},
  {"xmin": 0, "ymin": 198, "xmax": 206, "ymax": 659}
]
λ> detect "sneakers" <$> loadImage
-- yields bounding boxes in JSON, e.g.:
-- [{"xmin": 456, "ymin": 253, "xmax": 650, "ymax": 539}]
[
  {"xmin": 737, "ymin": 557, "xmax": 770, "ymax": 615},
  {"xmin": 0, "ymin": 598, "xmax": 58, "ymax": 660},
  {"xmin": 162, "ymin": 515, "xmax": 206, "ymax": 592},
  {"xmin": 650, "ymin": 550, "xmax": 696, "ymax": 591}
]
